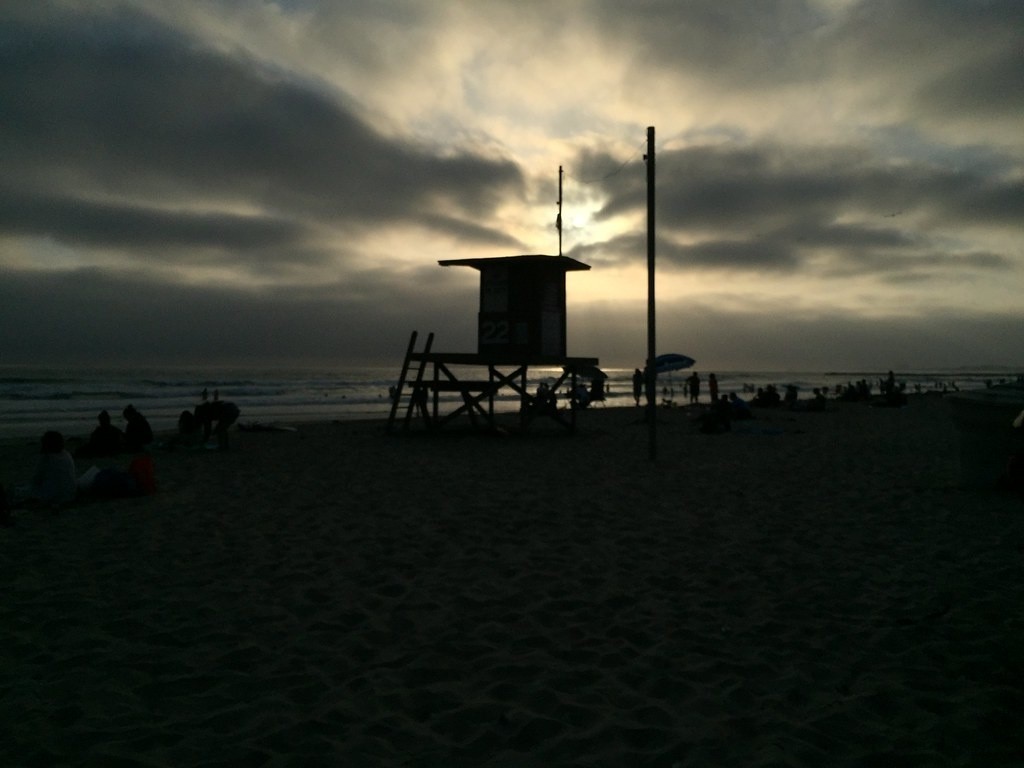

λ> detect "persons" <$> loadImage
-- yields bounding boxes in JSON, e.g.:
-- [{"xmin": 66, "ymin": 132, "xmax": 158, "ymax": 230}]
[
  {"xmin": 753, "ymin": 384, "xmax": 829, "ymax": 412},
  {"xmin": 708, "ymin": 373, "xmax": 718, "ymax": 401},
  {"xmin": 710, "ymin": 393, "xmax": 752, "ymax": 432},
  {"xmin": 914, "ymin": 383, "xmax": 960, "ymax": 391},
  {"xmin": 536, "ymin": 382, "xmax": 556, "ymax": 405},
  {"xmin": 37, "ymin": 431, "xmax": 75, "ymax": 490},
  {"xmin": 86, "ymin": 405, "xmax": 154, "ymax": 459},
  {"xmin": 686, "ymin": 372, "xmax": 700, "ymax": 403},
  {"xmin": 844, "ymin": 371, "xmax": 895, "ymax": 401},
  {"xmin": 389, "ymin": 386, "xmax": 428, "ymax": 417},
  {"xmin": 632, "ymin": 369, "xmax": 642, "ymax": 406},
  {"xmin": 179, "ymin": 387, "xmax": 240, "ymax": 450}
]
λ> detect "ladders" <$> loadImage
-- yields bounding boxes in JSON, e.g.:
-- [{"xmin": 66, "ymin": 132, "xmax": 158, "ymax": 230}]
[{"xmin": 388, "ymin": 330, "xmax": 435, "ymax": 420}]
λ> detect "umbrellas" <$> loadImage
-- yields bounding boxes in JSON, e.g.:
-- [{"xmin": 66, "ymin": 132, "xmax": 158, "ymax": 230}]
[
  {"xmin": 563, "ymin": 366, "xmax": 608, "ymax": 379},
  {"xmin": 656, "ymin": 353, "xmax": 696, "ymax": 400}
]
